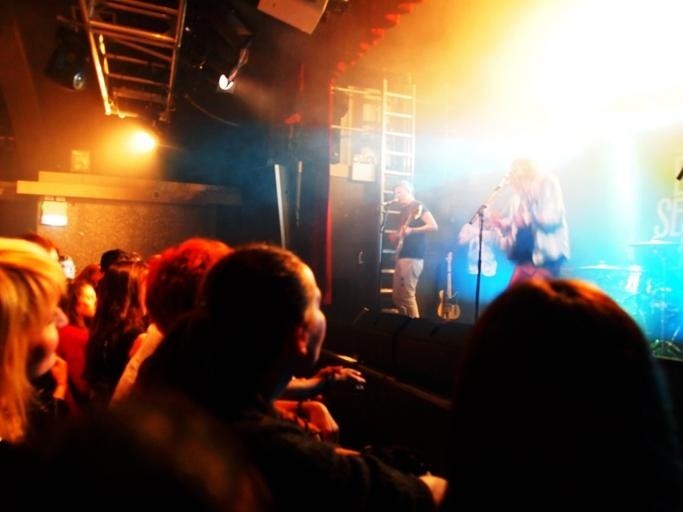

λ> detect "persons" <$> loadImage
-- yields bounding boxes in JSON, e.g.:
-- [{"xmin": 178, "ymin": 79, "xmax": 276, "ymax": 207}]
[
  {"xmin": 50, "ymin": 248, "xmax": 367, "ymax": 456},
  {"xmin": 488, "ymin": 158, "xmax": 573, "ymax": 288},
  {"xmin": 456, "ymin": 208, "xmax": 510, "ymax": 279},
  {"xmin": 385, "ymin": 182, "xmax": 437, "ymax": 318},
  {"xmin": 1, "ymin": 234, "xmax": 130, "ymax": 511},
  {"xmin": 101, "ymin": 243, "xmax": 447, "ymax": 510},
  {"xmin": 449, "ymin": 278, "xmax": 682, "ymax": 512}
]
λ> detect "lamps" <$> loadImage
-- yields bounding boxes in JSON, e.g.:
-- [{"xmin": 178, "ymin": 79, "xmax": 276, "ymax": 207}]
[{"xmin": 51, "ymin": 63, "xmax": 87, "ymax": 91}]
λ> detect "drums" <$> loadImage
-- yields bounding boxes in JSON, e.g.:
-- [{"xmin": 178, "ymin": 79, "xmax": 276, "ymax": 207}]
[{"xmin": 652, "ymin": 312, "xmax": 683, "ymax": 343}]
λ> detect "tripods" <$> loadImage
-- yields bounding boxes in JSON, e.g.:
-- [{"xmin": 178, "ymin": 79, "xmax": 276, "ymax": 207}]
[{"xmin": 648, "ymin": 249, "xmax": 682, "ymax": 359}]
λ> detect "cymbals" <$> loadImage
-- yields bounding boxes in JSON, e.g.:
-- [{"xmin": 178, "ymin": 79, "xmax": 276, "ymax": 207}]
[
  {"xmin": 623, "ymin": 239, "xmax": 679, "ymax": 246},
  {"xmin": 572, "ymin": 265, "xmax": 638, "ymax": 272}
]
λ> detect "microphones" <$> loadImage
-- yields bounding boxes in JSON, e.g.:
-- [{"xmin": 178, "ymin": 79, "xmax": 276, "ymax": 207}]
[{"xmin": 381, "ymin": 197, "xmax": 399, "ymax": 206}]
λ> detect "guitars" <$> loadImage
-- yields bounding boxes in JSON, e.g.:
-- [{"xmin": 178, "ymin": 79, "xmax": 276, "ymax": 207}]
[
  {"xmin": 391, "ymin": 203, "xmax": 422, "ymax": 259},
  {"xmin": 436, "ymin": 251, "xmax": 461, "ymax": 321}
]
